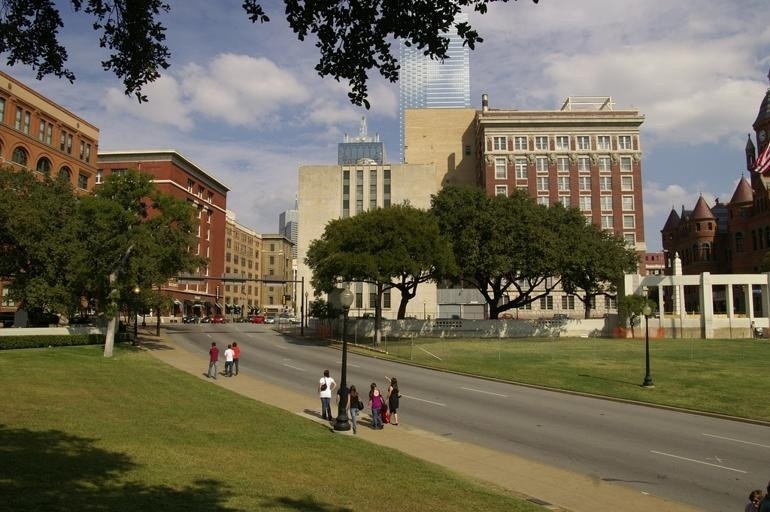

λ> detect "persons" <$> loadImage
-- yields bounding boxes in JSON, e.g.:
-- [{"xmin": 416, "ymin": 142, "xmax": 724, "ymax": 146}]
[
  {"xmin": 744, "ymin": 490, "xmax": 764, "ymax": 512},
  {"xmin": 336, "ymin": 382, "xmax": 351, "ymax": 407},
  {"xmin": 317, "ymin": 369, "xmax": 337, "ymax": 420},
  {"xmin": 224, "ymin": 344, "xmax": 235, "ymax": 378},
  {"xmin": 347, "ymin": 385, "xmax": 360, "ymax": 434},
  {"xmin": 231, "ymin": 342, "xmax": 240, "ymax": 376},
  {"xmin": 207, "ymin": 342, "xmax": 219, "ymax": 380},
  {"xmin": 369, "ymin": 383, "xmax": 384, "ymax": 429},
  {"xmin": 758, "ymin": 483, "xmax": 770, "ymax": 512},
  {"xmin": 384, "ymin": 376, "xmax": 400, "ymax": 425}
]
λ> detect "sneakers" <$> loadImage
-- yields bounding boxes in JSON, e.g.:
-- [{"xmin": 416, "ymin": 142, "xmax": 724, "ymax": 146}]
[
  {"xmin": 323, "ymin": 416, "xmax": 332, "ymax": 421},
  {"xmin": 393, "ymin": 423, "xmax": 398, "ymax": 425},
  {"xmin": 372, "ymin": 424, "xmax": 383, "ymax": 429}
]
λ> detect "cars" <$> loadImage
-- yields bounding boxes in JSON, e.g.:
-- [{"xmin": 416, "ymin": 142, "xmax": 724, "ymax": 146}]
[
  {"xmin": 181, "ymin": 314, "xmax": 228, "ymax": 323},
  {"xmin": 236, "ymin": 315, "xmax": 275, "ymax": 323},
  {"xmin": 0, "ymin": 306, "xmax": 95, "ymax": 326}
]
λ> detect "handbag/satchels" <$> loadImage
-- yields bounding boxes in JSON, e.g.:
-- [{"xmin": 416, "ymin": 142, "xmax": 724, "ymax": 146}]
[
  {"xmin": 321, "ymin": 384, "xmax": 327, "ymax": 391},
  {"xmin": 359, "ymin": 401, "xmax": 363, "ymax": 410},
  {"xmin": 383, "ymin": 413, "xmax": 390, "ymax": 423},
  {"xmin": 381, "ymin": 403, "xmax": 387, "ymax": 411}
]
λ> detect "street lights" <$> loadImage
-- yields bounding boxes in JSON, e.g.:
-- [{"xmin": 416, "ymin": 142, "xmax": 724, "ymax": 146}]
[
  {"xmin": 334, "ymin": 285, "xmax": 352, "ymax": 431},
  {"xmin": 305, "ymin": 291, "xmax": 308, "ymax": 328},
  {"xmin": 641, "ymin": 302, "xmax": 654, "ymax": 387},
  {"xmin": 132, "ymin": 287, "xmax": 140, "ymax": 346}
]
